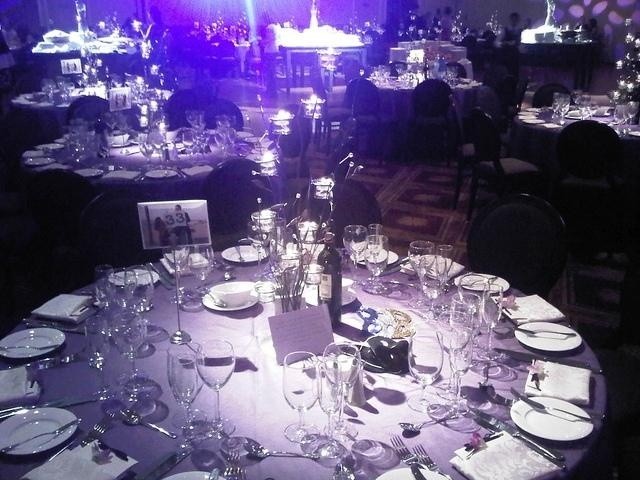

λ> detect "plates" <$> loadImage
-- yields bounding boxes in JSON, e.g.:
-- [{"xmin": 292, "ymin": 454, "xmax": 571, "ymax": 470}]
[
  {"xmin": 517, "ymin": 87, "xmax": 640, "ymax": 137},
  {"xmin": 0, "ymin": 204, "xmax": 596, "ymax": 480},
  {"xmin": 22, "ymin": 113, "xmax": 271, "ymax": 179}
]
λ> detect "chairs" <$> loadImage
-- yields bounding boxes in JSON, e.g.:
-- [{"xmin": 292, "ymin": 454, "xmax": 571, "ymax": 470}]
[
  {"xmin": 454, "ymin": 23, "xmax": 605, "ymax": 92},
  {"xmin": 11, "ymin": 30, "xmax": 312, "ymax": 253},
  {"xmin": 466, "ymin": 107, "xmax": 542, "ymax": 227},
  {"xmin": 528, "ymin": 83, "xmax": 578, "ymax": 109},
  {"xmin": 302, "ymin": 59, "xmax": 530, "ymax": 208},
  {"xmin": 555, "ymin": 120, "xmax": 624, "ymax": 252}
]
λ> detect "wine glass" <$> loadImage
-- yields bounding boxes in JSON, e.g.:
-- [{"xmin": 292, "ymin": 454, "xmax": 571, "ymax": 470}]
[
  {"xmin": 39, "ymin": 69, "xmax": 148, "ymax": 106},
  {"xmin": 367, "ymin": 63, "xmax": 428, "ymax": 89}
]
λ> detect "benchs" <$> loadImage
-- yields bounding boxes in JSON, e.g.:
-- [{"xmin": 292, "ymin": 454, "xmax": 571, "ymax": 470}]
[{"xmin": 456, "ymin": 193, "xmax": 568, "ymax": 305}]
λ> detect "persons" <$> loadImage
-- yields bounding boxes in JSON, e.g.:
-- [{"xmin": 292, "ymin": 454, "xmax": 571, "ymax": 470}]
[
  {"xmin": 154, "ymin": 217, "xmax": 170, "ymax": 246},
  {"xmin": 120, "ymin": 6, "xmax": 168, "ymax": 50},
  {"xmin": 494, "ymin": 12, "xmax": 524, "ymax": 84},
  {"xmin": 588, "ymin": 18, "xmax": 600, "ymax": 33},
  {"xmin": 403, "ymin": 4, "xmax": 422, "ymax": 29},
  {"xmin": 256, "ymin": 17, "xmax": 280, "ymax": 98},
  {"xmin": 172, "ymin": 205, "xmax": 192, "ymax": 245},
  {"xmin": 439, "ymin": 6, "xmax": 455, "ymax": 40}
]
